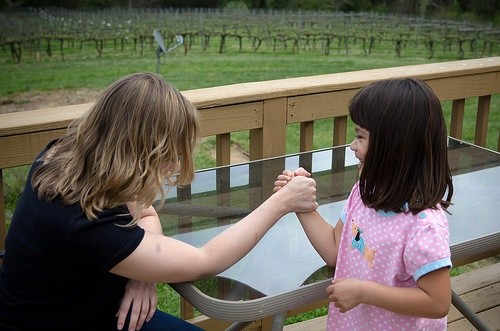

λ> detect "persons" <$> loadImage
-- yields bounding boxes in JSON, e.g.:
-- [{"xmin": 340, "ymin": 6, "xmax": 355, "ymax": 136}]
[
  {"xmin": 274, "ymin": 76, "xmax": 453, "ymax": 331},
  {"xmin": 0, "ymin": 73, "xmax": 318, "ymax": 331}
]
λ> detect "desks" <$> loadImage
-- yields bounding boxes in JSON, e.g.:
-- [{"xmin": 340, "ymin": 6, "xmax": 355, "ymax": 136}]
[{"xmin": 138, "ymin": 127, "xmax": 500, "ymax": 330}]
